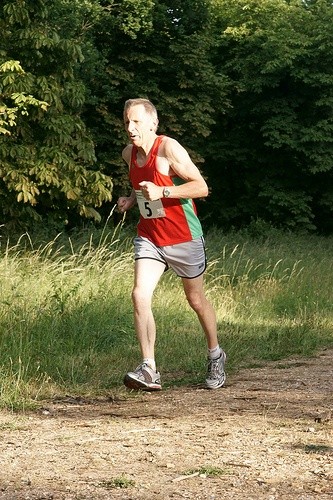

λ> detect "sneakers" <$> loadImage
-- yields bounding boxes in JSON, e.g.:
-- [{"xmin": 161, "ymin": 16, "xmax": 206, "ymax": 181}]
[
  {"xmin": 204, "ymin": 348, "xmax": 226, "ymax": 389},
  {"xmin": 123, "ymin": 363, "xmax": 162, "ymax": 391}
]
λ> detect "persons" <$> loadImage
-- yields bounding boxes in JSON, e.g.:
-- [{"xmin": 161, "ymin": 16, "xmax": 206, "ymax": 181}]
[{"xmin": 117, "ymin": 99, "xmax": 227, "ymax": 391}]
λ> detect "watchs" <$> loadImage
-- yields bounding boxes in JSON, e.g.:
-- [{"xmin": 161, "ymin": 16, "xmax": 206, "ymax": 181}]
[{"xmin": 163, "ymin": 186, "xmax": 170, "ymax": 198}]
